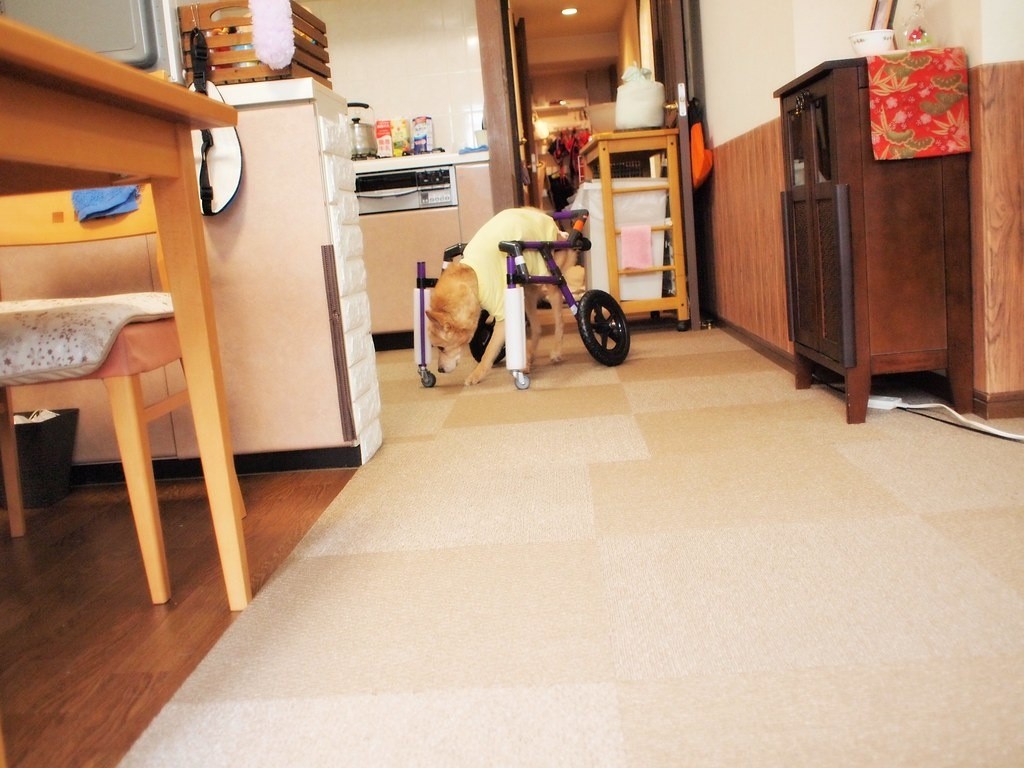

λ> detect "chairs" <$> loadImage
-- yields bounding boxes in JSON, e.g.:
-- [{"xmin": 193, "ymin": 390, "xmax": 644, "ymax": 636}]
[{"xmin": 0, "ymin": 68, "xmax": 247, "ymax": 605}]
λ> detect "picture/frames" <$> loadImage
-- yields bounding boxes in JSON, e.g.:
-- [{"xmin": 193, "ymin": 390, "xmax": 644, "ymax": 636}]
[{"xmin": 868, "ymin": 0, "xmax": 898, "ymax": 51}]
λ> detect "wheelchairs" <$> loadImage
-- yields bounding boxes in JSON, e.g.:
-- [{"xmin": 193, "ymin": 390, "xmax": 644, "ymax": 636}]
[{"xmin": 413, "ymin": 207, "xmax": 630, "ymax": 390}]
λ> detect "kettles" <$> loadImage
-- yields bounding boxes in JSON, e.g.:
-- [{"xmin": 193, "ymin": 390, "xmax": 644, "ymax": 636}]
[{"xmin": 346, "ymin": 102, "xmax": 378, "ymax": 155}]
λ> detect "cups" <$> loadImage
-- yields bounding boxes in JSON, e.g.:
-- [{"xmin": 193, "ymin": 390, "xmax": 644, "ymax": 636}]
[{"xmin": 474, "ymin": 130, "xmax": 489, "ymax": 149}]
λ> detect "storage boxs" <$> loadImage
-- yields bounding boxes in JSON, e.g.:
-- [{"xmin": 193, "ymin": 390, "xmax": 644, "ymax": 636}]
[
  {"xmin": 572, "ymin": 177, "xmax": 668, "ymax": 302},
  {"xmin": 178, "ymin": 0, "xmax": 332, "ymax": 90}
]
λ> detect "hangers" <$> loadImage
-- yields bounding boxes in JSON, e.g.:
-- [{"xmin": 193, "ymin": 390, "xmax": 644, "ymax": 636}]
[{"xmin": 548, "ymin": 164, "xmax": 568, "ymax": 179}]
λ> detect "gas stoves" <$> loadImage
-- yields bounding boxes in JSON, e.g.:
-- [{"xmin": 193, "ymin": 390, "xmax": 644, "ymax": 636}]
[{"xmin": 353, "ymin": 150, "xmax": 460, "ymax": 217}]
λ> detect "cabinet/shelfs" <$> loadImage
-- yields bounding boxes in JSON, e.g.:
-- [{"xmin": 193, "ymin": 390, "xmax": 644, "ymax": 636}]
[
  {"xmin": 579, "ymin": 128, "xmax": 689, "ymax": 332},
  {"xmin": 773, "ymin": 46, "xmax": 975, "ymax": 425},
  {"xmin": 358, "ymin": 206, "xmax": 462, "ymax": 354},
  {"xmin": 456, "ymin": 163, "xmax": 495, "ymax": 244}
]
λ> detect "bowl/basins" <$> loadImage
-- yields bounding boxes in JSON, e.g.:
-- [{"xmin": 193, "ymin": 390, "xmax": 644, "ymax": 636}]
[{"xmin": 849, "ymin": 29, "xmax": 896, "ymax": 58}]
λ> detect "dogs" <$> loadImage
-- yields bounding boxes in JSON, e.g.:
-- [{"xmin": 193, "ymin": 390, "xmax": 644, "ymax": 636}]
[{"xmin": 425, "ymin": 207, "xmax": 577, "ymax": 387}]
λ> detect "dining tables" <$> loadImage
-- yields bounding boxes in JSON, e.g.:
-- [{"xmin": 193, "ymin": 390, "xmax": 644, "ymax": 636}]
[{"xmin": 0, "ymin": 14, "xmax": 252, "ymax": 613}]
